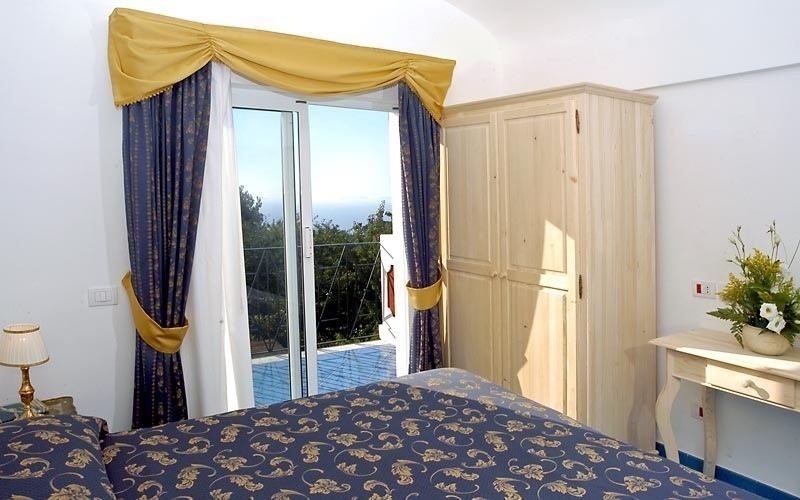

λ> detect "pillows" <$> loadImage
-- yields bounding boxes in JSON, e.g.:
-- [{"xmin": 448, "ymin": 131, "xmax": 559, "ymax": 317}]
[{"xmin": 1, "ymin": 409, "xmax": 120, "ymax": 500}]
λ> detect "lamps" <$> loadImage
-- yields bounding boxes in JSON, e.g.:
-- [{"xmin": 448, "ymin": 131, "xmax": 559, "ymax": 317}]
[{"xmin": 0, "ymin": 321, "xmax": 55, "ymax": 420}]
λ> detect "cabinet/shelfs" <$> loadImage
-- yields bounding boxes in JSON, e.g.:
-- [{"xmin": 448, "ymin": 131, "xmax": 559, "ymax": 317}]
[{"xmin": 437, "ymin": 77, "xmax": 667, "ymax": 461}]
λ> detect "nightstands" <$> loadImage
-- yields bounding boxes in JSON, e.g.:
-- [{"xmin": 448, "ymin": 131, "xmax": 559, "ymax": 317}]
[{"xmin": 37, "ymin": 395, "xmax": 80, "ymax": 417}]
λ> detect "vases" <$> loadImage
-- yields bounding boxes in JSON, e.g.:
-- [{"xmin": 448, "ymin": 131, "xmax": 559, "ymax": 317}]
[{"xmin": 740, "ymin": 322, "xmax": 795, "ymax": 359}]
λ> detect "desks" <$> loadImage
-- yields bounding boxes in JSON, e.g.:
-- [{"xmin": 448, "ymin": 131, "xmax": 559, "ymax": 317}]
[{"xmin": 643, "ymin": 323, "xmax": 800, "ymax": 480}]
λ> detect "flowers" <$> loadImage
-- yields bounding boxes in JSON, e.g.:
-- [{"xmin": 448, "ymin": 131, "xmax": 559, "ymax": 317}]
[{"xmin": 704, "ymin": 216, "xmax": 799, "ymax": 349}]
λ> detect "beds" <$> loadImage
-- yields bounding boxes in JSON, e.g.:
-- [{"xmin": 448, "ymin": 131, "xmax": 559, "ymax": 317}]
[{"xmin": 96, "ymin": 356, "xmax": 773, "ymax": 499}]
